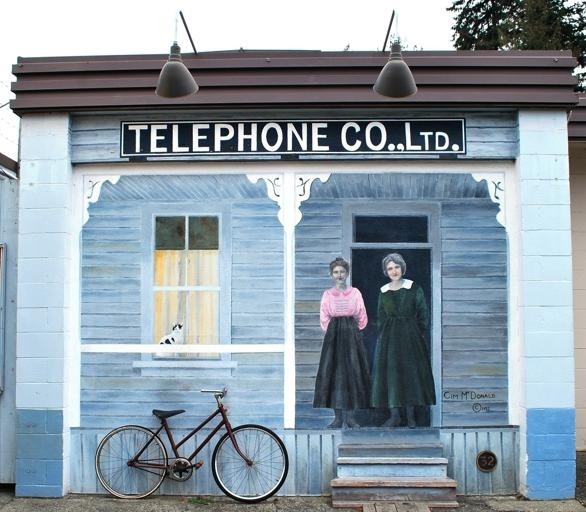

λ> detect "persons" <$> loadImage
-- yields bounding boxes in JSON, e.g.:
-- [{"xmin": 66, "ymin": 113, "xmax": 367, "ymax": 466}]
[
  {"xmin": 313, "ymin": 258, "xmax": 374, "ymax": 428},
  {"xmin": 369, "ymin": 253, "xmax": 435, "ymax": 429}
]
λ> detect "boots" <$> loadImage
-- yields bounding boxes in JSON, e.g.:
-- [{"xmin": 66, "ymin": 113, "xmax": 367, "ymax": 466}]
[
  {"xmin": 326, "ymin": 409, "xmax": 342, "ymax": 429},
  {"xmin": 405, "ymin": 407, "xmax": 416, "ymax": 429},
  {"xmin": 380, "ymin": 408, "xmax": 401, "ymax": 428},
  {"xmin": 346, "ymin": 409, "xmax": 360, "ymax": 428}
]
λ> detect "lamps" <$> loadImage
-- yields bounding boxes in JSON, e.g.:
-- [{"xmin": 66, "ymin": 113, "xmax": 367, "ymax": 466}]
[
  {"xmin": 154, "ymin": 12, "xmax": 199, "ymax": 98},
  {"xmin": 373, "ymin": 10, "xmax": 418, "ymax": 97}
]
